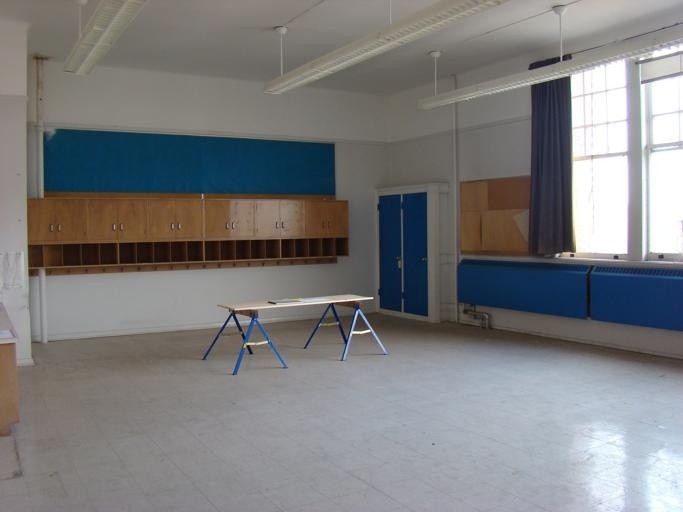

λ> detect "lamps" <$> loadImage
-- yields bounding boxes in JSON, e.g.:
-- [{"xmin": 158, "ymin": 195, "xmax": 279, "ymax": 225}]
[{"xmin": 61, "ymin": 0, "xmax": 145, "ymax": 77}]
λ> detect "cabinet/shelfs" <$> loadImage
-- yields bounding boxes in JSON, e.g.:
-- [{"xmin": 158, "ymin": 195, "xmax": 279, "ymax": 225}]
[
  {"xmin": 460, "ymin": 175, "xmax": 532, "ymax": 256},
  {"xmin": 374, "ymin": 183, "xmax": 448, "ymax": 323}
]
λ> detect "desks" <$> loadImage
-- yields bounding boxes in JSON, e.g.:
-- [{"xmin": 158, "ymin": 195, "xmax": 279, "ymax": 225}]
[{"xmin": 201, "ymin": 294, "xmax": 389, "ymax": 374}]
